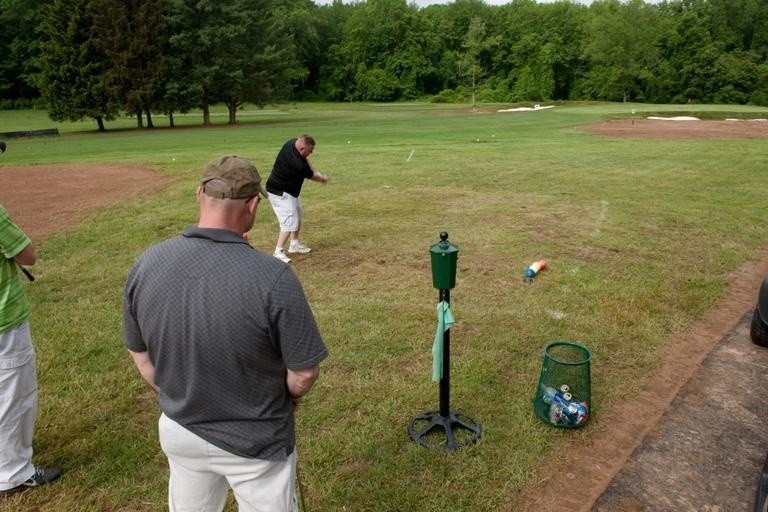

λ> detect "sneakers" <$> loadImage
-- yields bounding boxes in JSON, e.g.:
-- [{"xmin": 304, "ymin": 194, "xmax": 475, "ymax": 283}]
[
  {"xmin": 288, "ymin": 240, "xmax": 312, "ymax": 254},
  {"xmin": 0, "ymin": 467, "xmax": 63, "ymax": 498},
  {"xmin": 273, "ymin": 250, "xmax": 292, "ymax": 263}
]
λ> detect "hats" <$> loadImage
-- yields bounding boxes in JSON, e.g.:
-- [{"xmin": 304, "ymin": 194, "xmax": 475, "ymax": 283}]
[{"xmin": 201, "ymin": 155, "xmax": 268, "ymax": 199}]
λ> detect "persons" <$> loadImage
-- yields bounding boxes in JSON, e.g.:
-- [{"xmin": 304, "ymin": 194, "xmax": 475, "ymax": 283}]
[
  {"xmin": 265, "ymin": 134, "xmax": 331, "ymax": 264},
  {"xmin": 0, "ymin": 142, "xmax": 63, "ymax": 497},
  {"xmin": 123, "ymin": 153, "xmax": 328, "ymax": 512}
]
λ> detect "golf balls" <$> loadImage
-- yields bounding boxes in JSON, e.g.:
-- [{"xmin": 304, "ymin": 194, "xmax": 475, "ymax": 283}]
[
  {"xmin": 347, "ymin": 140, "xmax": 351, "ymax": 144},
  {"xmin": 476, "ymin": 138, "xmax": 479, "ymax": 141}
]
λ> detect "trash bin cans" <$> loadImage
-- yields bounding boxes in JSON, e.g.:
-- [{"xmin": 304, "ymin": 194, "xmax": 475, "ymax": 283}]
[{"xmin": 535, "ymin": 341, "xmax": 591, "ymax": 429}]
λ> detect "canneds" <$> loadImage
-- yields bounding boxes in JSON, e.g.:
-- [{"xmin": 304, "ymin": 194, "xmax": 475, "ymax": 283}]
[{"xmin": 542, "ymin": 384, "xmax": 589, "ymax": 425}]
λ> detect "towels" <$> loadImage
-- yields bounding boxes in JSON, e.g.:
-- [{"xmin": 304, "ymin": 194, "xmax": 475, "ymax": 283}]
[{"xmin": 432, "ymin": 300, "xmax": 455, "ymax": 382}]
